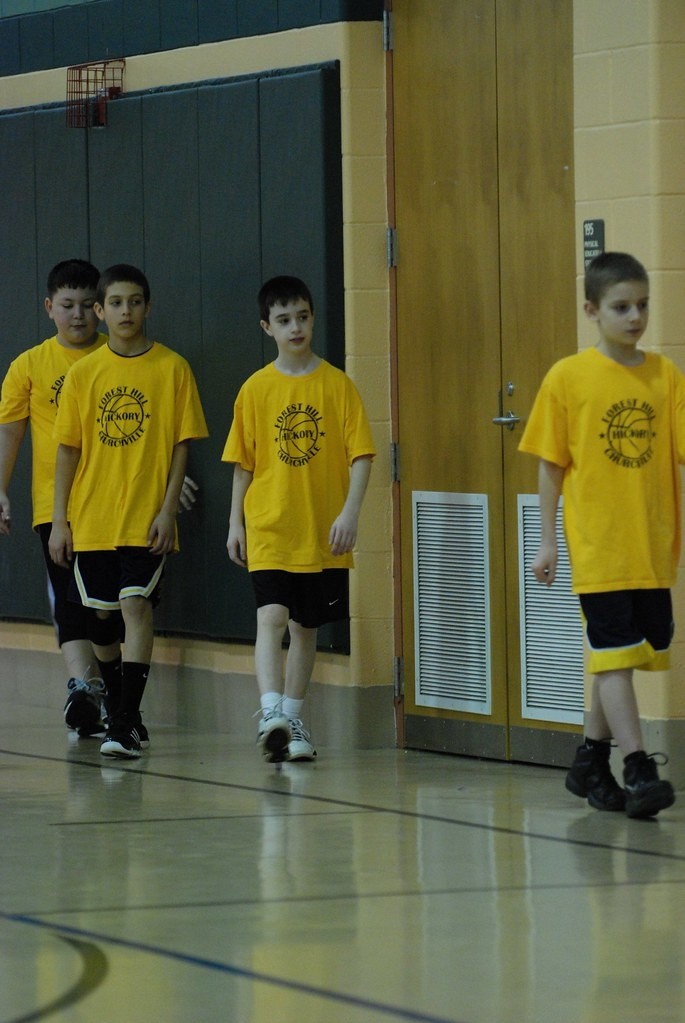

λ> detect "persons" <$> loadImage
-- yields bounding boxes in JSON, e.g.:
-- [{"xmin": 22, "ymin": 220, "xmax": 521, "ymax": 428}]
[
  {"xmin": 513, "ymin": 250, "xmax": 685, "ymax": 825},
  {"xmin": 0, "ymin": 260, "xmax": 201, "ymax": 745},
  {"xmin": 42, "ymin": 263, "xmax": 210, "ymax": 761},
  {"xmin": 220, "ymin": 275, "xmax": 377, "ymax": 765}
]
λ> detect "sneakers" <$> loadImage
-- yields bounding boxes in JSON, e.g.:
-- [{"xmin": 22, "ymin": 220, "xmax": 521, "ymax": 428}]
[
  {"xmin": 126, "ymin": 712, "xmax": 152, "ymax": 752},
  {"xmin": 99, "ymin": 715, "xmax": 142, "ymax": 759},
  {"xmin": 281, "ymin": 717, "xmax": 320, "ymax": 764},
  {"xmin": 60, "ymin": 665, "xmax": 109, "ymax": 738},
  {"xmin": 622, "ymin": 748, "xmax": 675, "ymax": 822},
  {"xmin": 563, "ymin": 737, "xmax": 627, "ymax": 812},
  {"xmin": 253, "ymin": 709, "xmax": 293, "ymax": 764}
]
